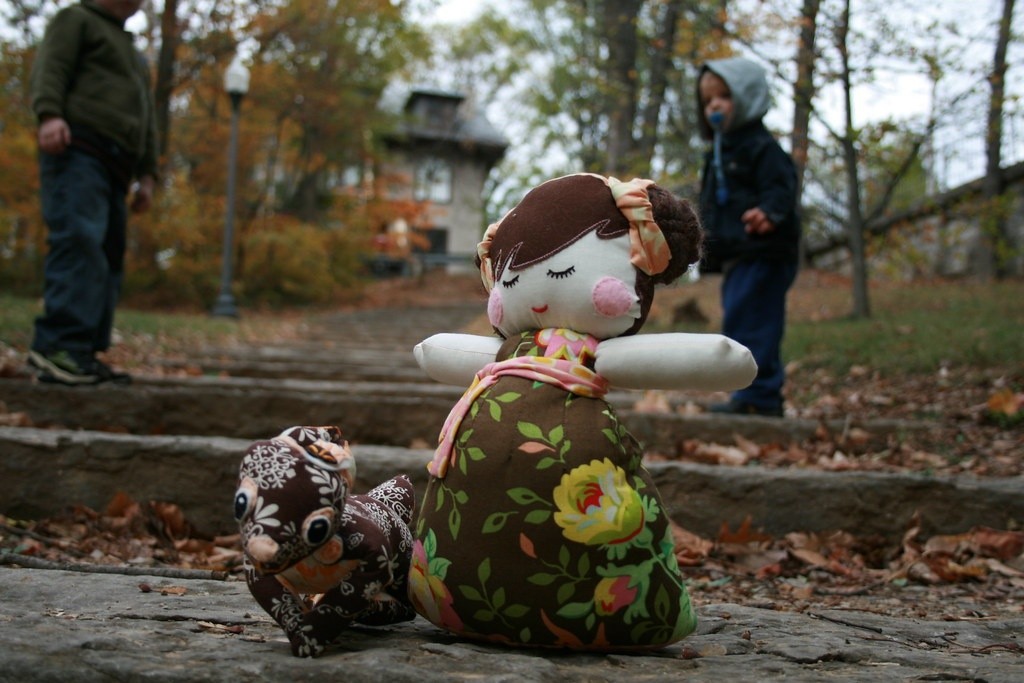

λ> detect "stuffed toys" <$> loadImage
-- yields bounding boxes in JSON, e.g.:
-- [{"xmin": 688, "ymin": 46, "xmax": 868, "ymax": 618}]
[{"xmin": 232, "ymin": 426, "xmax": 417, "ymax": 659}]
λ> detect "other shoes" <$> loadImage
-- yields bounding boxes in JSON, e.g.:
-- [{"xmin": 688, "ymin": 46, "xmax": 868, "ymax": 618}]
[{"xmin": 707, "ymin": 394, "xmax": 786, "ymax": 418}]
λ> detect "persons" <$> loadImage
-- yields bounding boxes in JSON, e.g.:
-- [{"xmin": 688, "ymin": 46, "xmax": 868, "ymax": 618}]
[
  {"xmin": 25, "ymin": 0, "xmax": 161, "ymax": 390},
  {"xmin": 409, "ymin": 172, "xmax": 759, "ymax": 651},
  {"xmin": 696, "ymin": 57, "xmax": 802, "ymax": 417}
]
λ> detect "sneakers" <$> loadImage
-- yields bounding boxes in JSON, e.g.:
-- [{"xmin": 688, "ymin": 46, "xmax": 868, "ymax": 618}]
[
  {"xmin": 29, "ymin": 339, "xmax": 96, "ymax": 384},
  {"xmin": 90, "ymin": 361, "xmax": 130, "ymax": 385}
]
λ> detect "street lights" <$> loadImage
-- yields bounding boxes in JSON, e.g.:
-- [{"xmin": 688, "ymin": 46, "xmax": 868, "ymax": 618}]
[{"xmin": 216, "ymin": 53, "xmax": 251, "ymax": 321}]
